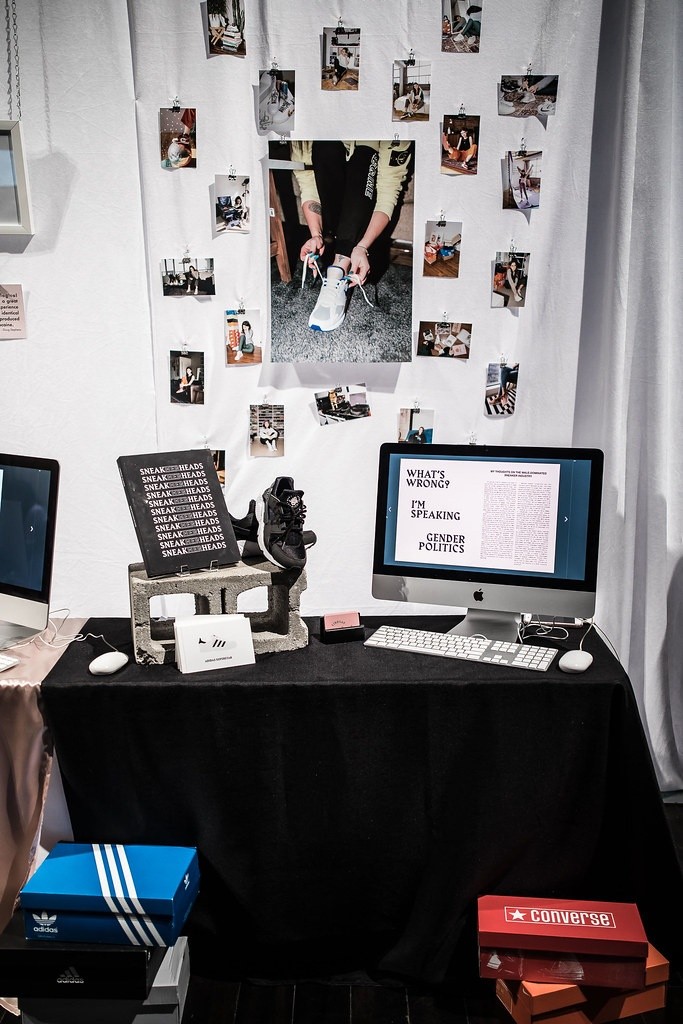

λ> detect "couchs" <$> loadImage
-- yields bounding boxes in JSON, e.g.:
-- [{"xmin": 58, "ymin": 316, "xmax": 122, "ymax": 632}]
[{"xmin": 178, "ymin": 271, "xmax": 215, "ymax": 292}]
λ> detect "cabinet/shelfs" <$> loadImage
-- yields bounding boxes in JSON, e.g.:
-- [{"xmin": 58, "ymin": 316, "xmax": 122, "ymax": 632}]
[
  {"xmin": 330, "ymin": 34, "xmax": 360, "ymax": 69},
  {"xmin": 172, "ymin": 357, "xmax": 191, "ymax": 379},
  {"xmin": 510, "ymin": 254, "xmax": 525, "ymax": 279},
  {"xmin": 250, "ymin": 405, "xmax": 284, "ymax": 439}
]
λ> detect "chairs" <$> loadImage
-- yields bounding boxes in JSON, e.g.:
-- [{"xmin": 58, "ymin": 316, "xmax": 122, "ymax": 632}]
[{"xmin": 500, "ymin": 363, "xmax": 519, "ymax": 391}]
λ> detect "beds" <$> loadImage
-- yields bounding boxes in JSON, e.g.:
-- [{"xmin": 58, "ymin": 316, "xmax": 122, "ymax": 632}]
[{"xmin": 393, "ymin": 91, "xmax": 429, "ymax": 114}]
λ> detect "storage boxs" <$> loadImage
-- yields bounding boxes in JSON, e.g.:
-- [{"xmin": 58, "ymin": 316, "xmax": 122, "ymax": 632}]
[
  {"xmin": 18, "ymin": 936, "xmax": 192, "ymax": 1024},
  {"xmin": 439, "ymin": 247, "xmax": 454, "ymax": 261},
  {"xmin": 424, "ymin": 243, "xmax": 437, "ymax": 265},
  {"xmin": 477, "ymin": 893, "xmax": 649, "ymax": 991},
  {"xmin": 0, "ymin": 905, "xmax": 170, "ymax": 1000},
  {"xmin": 445, "ymin": 240, "xmax": 453, "ymax": 246},
  {"xmin": 19, "ymin": 840, "xmax": 202, "ymax": 946},
  {"xmin": 443, "ymin": 246, "xmax": 453, "ymax": 250},
  {"xmin": 496, "ymin": 942, "xmax": 670, "ymax": 1024}
]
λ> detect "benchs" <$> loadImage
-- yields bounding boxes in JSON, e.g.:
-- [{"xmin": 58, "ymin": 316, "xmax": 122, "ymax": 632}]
[{"xmin": 405, "ymin": 429, "xmax": 432, "ymax": 444}]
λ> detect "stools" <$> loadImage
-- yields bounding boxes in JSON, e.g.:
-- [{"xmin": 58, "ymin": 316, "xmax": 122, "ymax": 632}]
[
  {"xmin": 269, "ymin": 158, "xmax": 305, "ymax": 283},
  {"xmin": 292, "ymin": 171, "xmax": 414, "ymax": 243},
  {"xmin": 209, "ymin": 27, "xmax": 226, "ymax": 45}
]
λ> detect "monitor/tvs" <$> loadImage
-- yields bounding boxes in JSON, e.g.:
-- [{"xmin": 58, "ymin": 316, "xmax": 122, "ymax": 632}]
[
  {"xmin": 372, "ymin": 442, "xmax": 605, "ymax": 643},
  {"xmin": 0, "ymin": 453, "xmax": 60, "ymax": 649}
]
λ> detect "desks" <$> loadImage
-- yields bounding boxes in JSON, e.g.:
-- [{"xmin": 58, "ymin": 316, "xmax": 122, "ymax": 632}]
[
  {"xmin": 40, "ymin": 614, "xmax": 683, "ymax": 1024},
  {"xmin": 0, "ymin": 618, "xmax": 89, "ymax": 940}
]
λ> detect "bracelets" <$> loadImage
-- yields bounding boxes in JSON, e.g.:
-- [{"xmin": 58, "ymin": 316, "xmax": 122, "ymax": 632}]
[{"xmin": 356, "ymin": 245, "xmax": 369, "ymax": 256}]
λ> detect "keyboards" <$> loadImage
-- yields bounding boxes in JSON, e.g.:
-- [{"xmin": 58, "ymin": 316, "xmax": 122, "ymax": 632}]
[
  {"xmin": 0, "ymin": 655, "xmax": 20, "ymax": 672},
  {"xmin": 363, "ymin": 626, "xmax": 558, "ymax": 672}
]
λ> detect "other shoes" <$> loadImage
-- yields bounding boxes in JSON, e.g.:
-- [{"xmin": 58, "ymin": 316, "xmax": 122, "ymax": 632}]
[
  {"xmin": 499, "ymin": 97, "xmax": 515, "ymax": 115},
  {"xmin": 232, "ymin": 346, "xmax": 238, "ymax": 350},
  {"xmin": 518, "ymin": 200, "xmax": 524, "ymax": 204},
  {"xmin": 468, "ymin": 35, "xmax": 476, "ymax": 44},
  {"xmin": 525, "ymin": 202, "xmax": 532, "ymax": 208},
  {"xmin": 489, "ymin": 397, "xmax": 508, "ymax": 407},
  {"xmin": 268, "ymin": 444, "xmax": 278, "ymax": 452},
  {"xmin": 186, "ymin": 289, "xmax": 191, "ymax": 292},
  {"xmin": 176, "ymin": 389, "xmax": 184, "ymax": 393},
  {"xmin": 193, "ymin": 289, "xmax": 199, "ymax": 295},
  {"xmin": 234, "ymin": 351, "xmax": 243, "ymax": 360},
  {"xmin": 461, "ymin": 164, "xmax": 468, "ymax": 170},
  {"xmin": 453, "ymin": 34, "xmax": 464, "ymax": 41}
]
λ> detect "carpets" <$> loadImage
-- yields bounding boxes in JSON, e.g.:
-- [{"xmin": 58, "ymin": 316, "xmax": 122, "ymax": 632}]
[
  {"xmin": 271, "ymin": 260, "xmax": 413, "ymax": 362},
  {"xmin": 441, "ymin": 157, "xmax": 476, "ymax": 174}
]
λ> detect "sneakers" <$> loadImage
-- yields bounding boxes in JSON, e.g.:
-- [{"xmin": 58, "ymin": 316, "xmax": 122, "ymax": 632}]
[
  {"xmin": 267, "ymin": 86, "xmax": 280, "ymax": 114},
  {"xmin": 400, "ymin": 111, "xmax": 412, "ymax": 119},
  {"xmin": 171, "ymin": 132, "xmax": 190, "ymax": 144},
  {"xmin": 538, "ymin": 97, "xmax": 556, "ymax": 115},
  {"xmin": 520, "ymin": 92, "xmax": 536, "ymax": 103},
  {"xmin": 272, "ymin": 98, "xmax": 295, "ymax": 124},
  {"xmin": 514, "ymin": 294, "xmax": 523, "ymax": 302},
  {"xmin": 307, "ymin": 266, "xmax": 349, "ymax": 331},
  {"xmin": 255, "ymin": 477, "xmax": 307, "ymax": 572}
]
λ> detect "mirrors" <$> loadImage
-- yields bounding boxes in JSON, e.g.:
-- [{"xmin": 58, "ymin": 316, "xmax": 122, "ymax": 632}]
[{"xmin": 449, "ymin": 0, "xmax": 470, "ymax": 35}]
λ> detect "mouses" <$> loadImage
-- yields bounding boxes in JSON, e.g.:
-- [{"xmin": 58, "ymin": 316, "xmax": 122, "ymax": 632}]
[
  {"xmin": 89, "ymin": 652, "xmax": 128, "ymax": 675},
  {"xmin": 559, "ymin": 650, "xmax": 594, "ymax": 673}
]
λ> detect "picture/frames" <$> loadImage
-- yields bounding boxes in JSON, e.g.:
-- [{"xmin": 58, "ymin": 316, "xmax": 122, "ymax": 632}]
[
  {"xmin": 436, "ymin": 233, "xmax": 443, "ymax": 244},
  {"xmin": 0, "ymin": 120, "xmax": 34, "ymax": 235},
  {"xmin": 429, "ymin": 232, "xmax": 438, "ymax": 245}
]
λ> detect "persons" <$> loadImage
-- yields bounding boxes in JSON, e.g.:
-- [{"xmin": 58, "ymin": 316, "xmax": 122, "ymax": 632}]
[
  {"xmin": 185, "ymin": 266, "xmax": 199, "ymax": 294},
  {"xmin": 230, "ymin": 196, "xmax": 247, "ymax": 228},
  {"xmin": 209, "ymin": 7, "xmax": 226, "ymax": 27},
  {"xmin": 442, "ymin": 127, "xmax": 477, "ymax": 169},
  {"xmin": 504, "ymin": 261, "xmax": 527, "ymax": 301},
  {"xmin": 171, "ymin": 108, "xmax": 196, "ymax": 143},
  {"xmin": 260, "ymin": 420, "xmax": 278, "ymax": 452},
  {"xmin": 176, "ymin": 367, "xmax": 195, "ymax": 393},
  {"xmin": 520, "ymin": 76, "xmax": 557, "ymax": 93},
  {"xmin": 332, "ymin": 47, "xmax": 350, "ymax": 85},
  {"xmin": 400, "ymin": 82, "xmax": 425, "ymax": 120},
  {"xmin": 232, "ymin": 321, "xmax": 254, "ymax": 360},
  {"xmin": 291, "ymin": 140, "xmax": 411, "ymax": 330},
  {"xmin": 454, "ymin": 15, "xmax": 466, "ymax": 31},
  {"xmin": 489, "ymin": 363, "xmax": 518, "ymax": 407},
  {"xmin": 408, "ymin": 427, "xmax": 426, "ymax": 444},
  {"xmin": 454, "ymin": 6, "xmax": 482, "ymax": 44},
  {"xmin": 516, "ymin": 164, "xmax": 535, "ymax": 206}
]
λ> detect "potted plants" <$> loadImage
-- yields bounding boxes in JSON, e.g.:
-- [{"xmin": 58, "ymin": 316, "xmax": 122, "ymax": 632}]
[{"xmin": 207, "ymin": 0, "xmax": 228, "ymax": 28}]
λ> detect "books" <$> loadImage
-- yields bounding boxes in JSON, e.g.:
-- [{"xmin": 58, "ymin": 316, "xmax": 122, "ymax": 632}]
[{"xmin": 222, "ymin": 26, "xmax": 242, "ymax": 52}]
